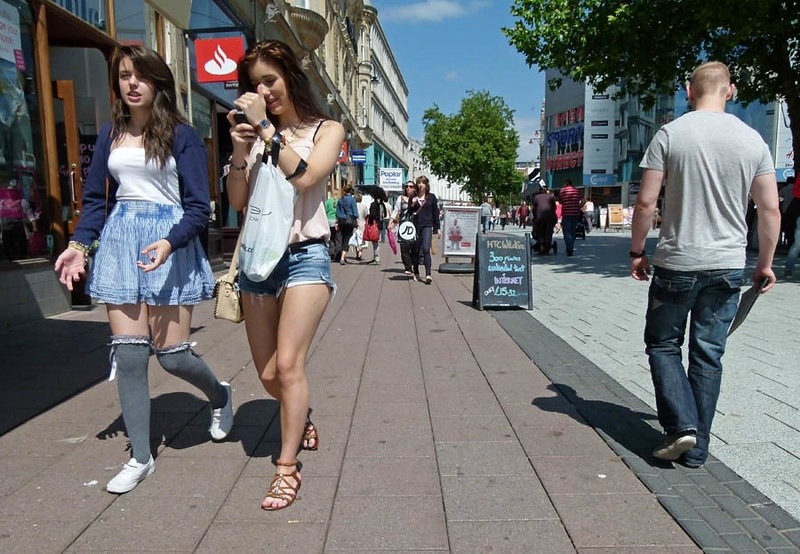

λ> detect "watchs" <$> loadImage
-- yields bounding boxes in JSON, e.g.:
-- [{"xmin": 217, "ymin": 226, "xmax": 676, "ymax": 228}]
[{"xmin": 254, "ymin": 118, "xmax": 271, "ymax": 131}]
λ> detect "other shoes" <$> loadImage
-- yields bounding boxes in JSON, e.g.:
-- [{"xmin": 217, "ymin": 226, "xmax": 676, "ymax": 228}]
[
  {"xmin": 341, "ymin": 260, "xmax": 346, "ymax": 265},
  {"xmin": 539, "ymin": 251, "xmax": 548, "ymax": 256},
  {"xmin": 367, "ymin": 257, "xmax": 380, "ymax": 265},
  {"xmin": 784, "ymin": 274, "xmax": 794, "ymax": 280},
  {"xmin": 414, "ymin": 274, "xmax": 421, "ymax": 281},
  {"xmin": 567, "ymin": 253, "xmax": 573, "ymax": 256},
  {"xmin": 534, "ymin": 243, "xmax": 541, "ymax": 252},
  {"xmin": 356, "ymin": 256, "xmax": 362, "ymax": 261},
  {"xmin": 426, "ymin": 276, "xmax": 432, "ymax": 285},
  {"xmin": 405, "ymin": 271, "xmax": 411, "ymax": 274}
]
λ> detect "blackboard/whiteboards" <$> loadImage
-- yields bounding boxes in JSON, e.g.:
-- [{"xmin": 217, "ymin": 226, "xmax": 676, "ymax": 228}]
[{"xmin": 473, "ymin": 232, "xmax": 533, "ymax": 306}]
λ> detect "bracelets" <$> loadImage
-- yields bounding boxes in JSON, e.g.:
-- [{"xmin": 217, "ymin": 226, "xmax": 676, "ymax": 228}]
[
  {"xmin": 69, "ymin": 239, "xmax": 100, "ymax": 258},
  {"xmin": 260, "ymin": 129, "xmax": 282, "ymax": 168},
  {"xmin": 630, "ymin": 250, "xmax": 645, "ymax": 257}
]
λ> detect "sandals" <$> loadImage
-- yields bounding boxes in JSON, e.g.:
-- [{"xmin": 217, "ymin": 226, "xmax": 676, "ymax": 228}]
[
  {"xmin": 263, "ymin": 461, "xmax": 301, "ymax": 510},
  {"xmin": 300, "ymin": 421, "xmax": 319, "ymax": 451}
]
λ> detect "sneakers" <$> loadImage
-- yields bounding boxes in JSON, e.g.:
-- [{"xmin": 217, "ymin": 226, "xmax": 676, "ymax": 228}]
[
  {"xmin": 106, "ymin": 454, "xmax": 155, "ymax": 493},
  {"xmin": 685, "ymin": 458, "xmax": 704, "ymax": 469},
  {"xmin": 208, "ymin": 382, "xmax": 233, "ymax": 440},
  {"xmin": 652, "ymin": 430, "xmax": 697, "ymax": 460}
]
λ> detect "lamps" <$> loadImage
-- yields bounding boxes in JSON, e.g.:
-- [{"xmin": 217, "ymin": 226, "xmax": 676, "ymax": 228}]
[
  {"xmin": 358, "ymin": 60, "xmax": 380, "ymax": 84},
  {"xmin": 382, "ymin": 112, "xmax": 399, "ymax": 129},
  {"xmin": 403, "ymin": 140, "xmax": 414, "ymax": 151}
]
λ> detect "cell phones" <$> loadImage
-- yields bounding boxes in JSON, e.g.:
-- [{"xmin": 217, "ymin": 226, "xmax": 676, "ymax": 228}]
[{"xmin": 232, "ymin": 112, "xmax": 249, "ymax": 124}]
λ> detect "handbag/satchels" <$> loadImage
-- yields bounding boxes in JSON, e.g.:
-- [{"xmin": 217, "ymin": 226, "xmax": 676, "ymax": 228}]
[
  {"xmin": 239, "ymin": 132, "xmax": 308, "ymax": 284},
  {"xmin": 355, "ymin": 227, "xmax": 368, "ymax": 251},
  {"xmin": 388, "ymin": 229, "xmax": 397, "ymax": 254},
  {"xmin": 397, "ymin": 215, "xmax": 416, "ymax": 244},
  {"xmin": 346, "ymin": 215, "xmax": 358, "ymax": 229},
  {"xmin": 211, "ymin": 271, "xmax": 244, "ymax": 323},
  {"xmin": 348, "ymin": 233, "xmax": 357, "ymax": 247},
  {"xmin": 363, "ymin": 215, "xmax": 379, "ymax": 241}
]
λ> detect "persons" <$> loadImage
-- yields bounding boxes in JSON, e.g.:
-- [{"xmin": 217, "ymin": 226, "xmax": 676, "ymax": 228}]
[
  {"xmin": 746, "ymin": 171, "xmax": 800, "ymax": 274},
  {"xmin": 53, "ymin": 46, "xmax": 234, "ymax": 495},
  {"xmin": 628, "ymin": 59, "xmax": 781, "ymax": 469},
  {"xmin": 480, "ymin": 179, "xmax": 595, "ymax": 256},
  {"xmin": 221, "ymin": 35, "xmax": 442, "ymax": 517},
  {"xmin": 0, "ymin": 175, "xmax": 38, "ymax": 261},
  {"xmin": 652, "ymin": 203, "xmax": 662, "ymax": 230},
  {"xmin": 627, "ymin": 204, "xmax": 636, "ymax": 230}
]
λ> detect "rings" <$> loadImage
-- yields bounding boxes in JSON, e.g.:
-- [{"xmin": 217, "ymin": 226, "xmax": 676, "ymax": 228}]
[{"xmin": 240, "ymin": 131, "xmax": 245, "ymax": 138}]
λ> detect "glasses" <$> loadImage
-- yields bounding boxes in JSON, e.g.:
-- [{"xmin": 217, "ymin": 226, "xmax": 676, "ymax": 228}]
[{"xmin": 405, "ymin": 185, "xmax": 414, "ymax": 188}]
